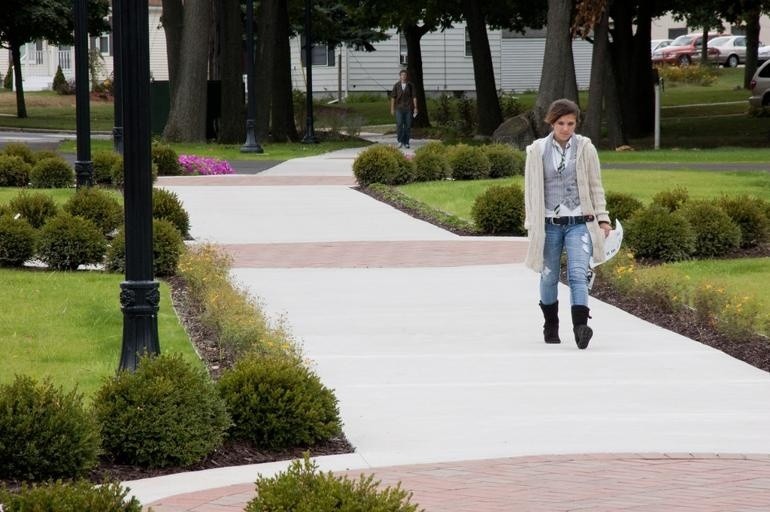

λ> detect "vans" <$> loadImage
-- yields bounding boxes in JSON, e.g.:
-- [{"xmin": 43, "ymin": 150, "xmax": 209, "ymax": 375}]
[{"xmin": 650, "ymin": 38, "xmax": 674, "ymax": 56}]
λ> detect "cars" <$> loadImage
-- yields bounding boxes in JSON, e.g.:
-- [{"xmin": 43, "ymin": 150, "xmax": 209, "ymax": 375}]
[{"xmin": 697, "ymin": 35, "xmax": 770, "ymax": 68}]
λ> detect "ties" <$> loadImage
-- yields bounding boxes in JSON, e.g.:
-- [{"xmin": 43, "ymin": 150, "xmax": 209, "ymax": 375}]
[{"xmin": 554, "ymin": 145, "xmax": 570, "ymax": 215}]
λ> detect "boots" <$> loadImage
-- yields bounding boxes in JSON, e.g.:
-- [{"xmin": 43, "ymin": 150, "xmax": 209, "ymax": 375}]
[
  {"xmin": 572, "ymin": 305, "xmax": 593, "ymax": 349},
  {"xmin": 539, "ymin": 300, "xmax": 560, "ymax": 343}
]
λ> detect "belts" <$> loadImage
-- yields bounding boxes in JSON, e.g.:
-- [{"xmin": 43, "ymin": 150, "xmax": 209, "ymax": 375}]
[{"xmin": 545, "ymin": 215, "xmax": 594, "ymax": 226}]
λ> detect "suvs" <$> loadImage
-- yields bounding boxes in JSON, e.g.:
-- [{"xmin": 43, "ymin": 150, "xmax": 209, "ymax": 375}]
[
  {"xmin": 749, "ymin": 57, "xmax": 770, "ymax": 116},
  {"xmin": 652, "ymin": 31, "xmax": 734, "ymax": 66}
]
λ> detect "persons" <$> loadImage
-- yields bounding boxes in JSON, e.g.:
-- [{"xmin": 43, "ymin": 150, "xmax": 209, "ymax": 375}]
[
  {"xmin": 524, "ymin": 99, "xmax": 613, "ymax": 349},
  {"xmin": 390, "ymin": 69, "xmax": 419, "ymax": 149}
]
